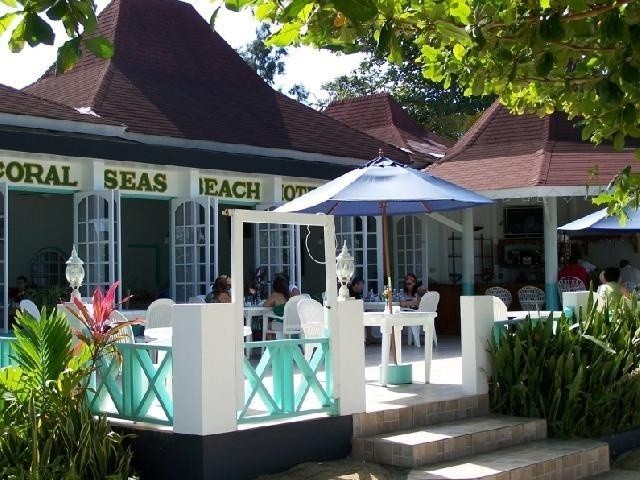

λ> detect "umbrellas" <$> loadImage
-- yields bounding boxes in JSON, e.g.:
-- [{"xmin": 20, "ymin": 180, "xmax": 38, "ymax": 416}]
[
  {"xmin": 273, "ymin": 148, "xmax": 495, "ymax": 364},
  {"xmin": 557, "ymin": 196, "xmax": 640, "ymax": 234}
]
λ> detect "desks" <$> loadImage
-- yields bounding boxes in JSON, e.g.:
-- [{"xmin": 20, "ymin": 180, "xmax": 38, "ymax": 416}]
[{"xmin": 244, "ymin": 306, "xmax": 272, "ymax": 360}]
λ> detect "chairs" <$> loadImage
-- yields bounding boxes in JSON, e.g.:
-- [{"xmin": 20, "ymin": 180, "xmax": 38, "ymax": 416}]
[
  {"xmin": 22, "ymin": 294, "xmax": 175, "ymax": 407},
  {"xmin": 262, "ymin": 287, "xmax": 440, "ymax": 387},
  {"xmin": 485, "ymin": 275, "xmax": 587, "ymax": 311}
]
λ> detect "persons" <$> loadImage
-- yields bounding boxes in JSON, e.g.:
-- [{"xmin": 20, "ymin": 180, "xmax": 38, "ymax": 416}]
[
  {"xmin": 558, "ymin": 255, "xmax": 640, "ymax": 321},
  {"xmin": 205, "ymin": 272, "xmax": 300, "ymax": 340},
  {"xmin": 9, "ymin": 276, "xmax": 34, "ymax": 302},
  {"xmin": 400, "ymin": 286, "xmax": 427, "ymax": 309},
  {"xmin": 348, "ymin": 277, "xmax": 382, "ymax": 345},
  {"xmin": 402, "ymin": 274, "xmax": 420, "ymax": 297}
]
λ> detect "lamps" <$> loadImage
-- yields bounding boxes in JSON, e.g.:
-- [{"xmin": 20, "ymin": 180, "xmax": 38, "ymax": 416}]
[
  {"xmin": 335, "ymin": 241, "xmax": 359, "ymax": 299},
  {"xmin": 64, "ymin": 245, "xmax": 87, "ymax": 304}
]
